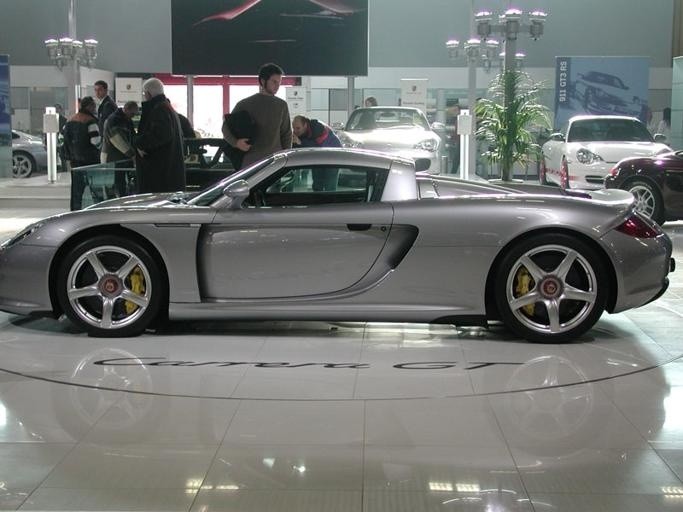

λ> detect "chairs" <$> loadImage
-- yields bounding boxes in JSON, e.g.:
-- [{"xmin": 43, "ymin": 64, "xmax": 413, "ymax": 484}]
[
  {"xmin": 399, "ymin": 116, "xmax": 413, "ymax": 126},
  {"xmin": 363, "ymin": 171, "xmax": 377, "ymax": 202},
  {"xmin": 607, "ymin": 124, "xmax": 638, "ymax": 140},
  {"xmin": 354, "ymin": 114, "xmax": 378, "ymax": 129},
  {"xmin": 571, "ymin": 126, "xmax": 592, "ymax": 142}
]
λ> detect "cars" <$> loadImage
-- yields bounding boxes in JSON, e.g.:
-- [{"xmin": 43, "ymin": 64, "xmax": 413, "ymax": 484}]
[
  {"xmin": 569, "ymin": 70, "xmax": 644, "ymax": 122},
  {"xmin": 9, "ymin": 129, "xmax": 61, "ymax": 178},
  {"xmin": 65, "ymin": 138, "xmax": 312, "ymax": 210},
  {"xmin": 603, "ymin": 149, "xmax": 683, "ymax": 228}
]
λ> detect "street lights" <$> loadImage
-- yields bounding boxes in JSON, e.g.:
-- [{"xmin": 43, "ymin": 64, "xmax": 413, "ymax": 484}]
[
  {"xmin": 444, "ymin": 39, "xmax": 499, "ymax": 188},
  {"xmin": 43, "ymin": 36, "xmax": 99, "ymax": 117},
  {"xmin": 477, "ymin": 51, "xmax": 529, "ymax": 135},
  {"xmin": 474, "ymin": 5, "xmax": 546, "ymax": 181}
]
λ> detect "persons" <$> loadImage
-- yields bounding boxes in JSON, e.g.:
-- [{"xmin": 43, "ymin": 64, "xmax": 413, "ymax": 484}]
[
  {"xmin": 657, "ymin": 107, "xmax": 671, "ymax": 146},
  {"xmin": 166, "ymin": 98, "xmax": 199, "ymax": 161},
  {"xmin": 365, "ymin": 96, "xmax": 377, "ymax": 107},
  {"xmin": 44, "ymin": 103, "xmax": 68, "ymax": 172},
  {"xmin": 221, "ymin": 62, "xmax": 293, "ymax": 171},
  {"xmin": 133, "ymin": 77, "xmax": 186, "ymax": 194},
  {"xmin": 292, "ymin": 115, "xmax": 342, "ymax": 148},
  {"xmin": 99, "ymin": 101, "xmax": 138, "ymax": 198},
  {"xmin": 94, "ymin": 80, "xmax": 118, "ymax": 138},
  {"xmin": 63, "ymin": 96, "xmax": 103, "ymax": 209}
]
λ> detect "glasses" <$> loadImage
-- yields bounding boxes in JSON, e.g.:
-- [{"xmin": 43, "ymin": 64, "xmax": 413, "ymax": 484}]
[
  {"xmin": 141, "ymin": 91, "xmax": 147, "ymax": 95},
  {"xmin": 270, "ymin": 79, "xmax": 281, "ymax": 86}
]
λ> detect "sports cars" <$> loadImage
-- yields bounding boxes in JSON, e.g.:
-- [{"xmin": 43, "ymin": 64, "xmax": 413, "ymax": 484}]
[
  {"xmin": 540, "ymin": 107, "xmax": 676, "ymax": 197},
  {"xmin": 0, "ymin": 147, "xmax": 677, "ymax": 343},
  {"xmin": 329, "ymin": 105, "xmax": 446, "ymax": 178}
]
